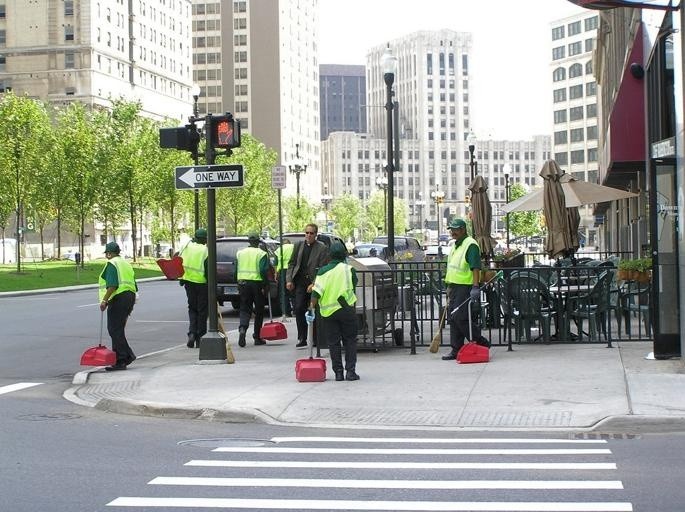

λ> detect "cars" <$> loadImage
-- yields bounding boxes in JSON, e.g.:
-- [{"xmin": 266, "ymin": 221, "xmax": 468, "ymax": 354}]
[{"xmin": 349, "ymin": 243, "xmax": 400, "ymax": 261}]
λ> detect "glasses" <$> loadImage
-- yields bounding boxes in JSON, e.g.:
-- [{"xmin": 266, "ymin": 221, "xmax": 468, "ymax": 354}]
[{"xmin": 306, "ymin": 231, "xmax": 314, "ymax": 234}]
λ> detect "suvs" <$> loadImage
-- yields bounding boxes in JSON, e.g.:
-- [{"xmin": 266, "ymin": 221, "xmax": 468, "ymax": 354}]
[
  {"xmin": 272, "ymin": 231, "xmax": 357, "ymax": 257},
  {"xmin": 208, "ymin": 233, "xmax": 294, "ymax": 317}
]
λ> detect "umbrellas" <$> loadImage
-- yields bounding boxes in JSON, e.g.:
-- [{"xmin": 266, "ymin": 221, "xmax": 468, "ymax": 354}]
[
  {"xmin": 468, "ymin": 175, "xmax": 498, "ymax": 270},
  {"xmin": 499, "ymin": 159, "xmax": 640, "ymax": 287}
]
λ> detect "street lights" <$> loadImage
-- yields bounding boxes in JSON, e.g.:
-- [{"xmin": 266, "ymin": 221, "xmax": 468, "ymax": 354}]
[
  {"xmin": 415, "ymin": 192, "xmax": 427, "ymax": 233},
  {"xmin": 432, "ymin": 176, "xmax": 448, "ymax": 244},
  {"xmin": 501, "ymin": 159, "xmax": 514, "ymax": 249},
  {"xmin": 379, "ymin": 38, "xmax": 400, "ymax": 260},
  {"xmin": 377, "ymin": 158, "xmax": 390, "ymax": 235},
  {"xmin": 319, "ymin": 180, "xmax": 335, "ymax": 226},
  {"xmin": 188, "ymin": 79, "xmax": 204, "ymax": 234},
  {"xmin": 288, "ymin": 137, "xmax": 310, "ymax": 211},
  {"xmin": 466, "ymin": 123, "xmax": 481, "ymax": 186}
]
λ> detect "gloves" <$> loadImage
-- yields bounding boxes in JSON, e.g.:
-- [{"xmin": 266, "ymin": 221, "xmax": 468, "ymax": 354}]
[
  {"xmin": 305, "ymin": 308, "xmax": 316, "ymax": 325},
  {"xmin": 470, "ymin": 286, "xmax": 480, "ymax": 303}
]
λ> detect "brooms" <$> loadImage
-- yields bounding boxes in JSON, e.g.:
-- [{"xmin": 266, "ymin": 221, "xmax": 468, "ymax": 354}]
[
  {"xmin": 213, "ymin": 300, "xmax": 236, "ymax": 365},
  {"xmin": 427, "ymin": 296, "xmax": 449, "ymax": 353}
]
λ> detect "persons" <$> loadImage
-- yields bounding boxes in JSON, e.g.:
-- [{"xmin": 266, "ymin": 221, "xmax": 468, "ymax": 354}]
[
  {"xmin": 177, "ymin": 229, "xmax": 208, "ymax": 348},
  {"xmin": 305, "ymin": 240, "xmax": 360, "ymax": 382},
  {"xmin": 234, "ymin": 230, "xmax": 275, "ymax": 347},
  {"xmin": 441, "ymin": 218, "xmax": 491, "ymax": 361},
  {"xmin": 273, "ymin": 238, "xmax": 297, "ymax": 317},
  {"xmin": 98, "ymin": 241, "xmax": 138, "ymax": 371},
  {"xmin": 285, "ymin": 223, "xmax": 329, "ymax": 348}
]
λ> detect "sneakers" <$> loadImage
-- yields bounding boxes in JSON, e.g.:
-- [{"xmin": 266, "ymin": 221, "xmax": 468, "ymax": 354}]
[
  {"xmin": 254, "ymin": 338, "xmax": 266, "ymax": 345},
  {"xmin": 105, "ymin": 349, "xmax": 136, "ymax": 371},
  {"xmin": 187, "ymin": 332, "xmax": 206, "ymax": 348},
  {"xmin": 335, "ymin": 368, "xmax": 360, "ymax": 381},
  {"xmin": 238, "ymin": 326, "xmax": 246, "ymax": 347},
  {"xmin": 477, "ymin": 336, "xmax": 491, "ymax": 348},
  {"xmin": 442, "ymin": 348, "xmax": 458, "ymax": 360}
]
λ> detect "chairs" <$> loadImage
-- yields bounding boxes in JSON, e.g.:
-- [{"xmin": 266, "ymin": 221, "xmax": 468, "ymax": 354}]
[{"xmin": 422, "ymin": 255, "xmax": 653, "ymax": 344}]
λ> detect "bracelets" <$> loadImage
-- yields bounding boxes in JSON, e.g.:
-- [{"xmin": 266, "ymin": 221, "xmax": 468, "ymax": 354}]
[{"xmin": 102, "ymin": 299, "xmax": 107, "ymax": 304}]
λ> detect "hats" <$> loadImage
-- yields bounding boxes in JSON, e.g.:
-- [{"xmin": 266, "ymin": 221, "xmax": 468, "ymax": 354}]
[
  {"xmin": 195, "ymin": 229, "xmax": 207, "ymax": 238},
  {"xmin": 247, "ymin": 234, "xmax": 260, "ymax": 240},
  {"xmin": 104, "ymin": 242, "xmax": 121, "ymax": 253},
  {"xmin": 330, "ymin": 242, "xmax": 345, "ymax": 256},
  {"xmin": 447, "ymin": 219, "xmax": 466, "ymax": 230}
]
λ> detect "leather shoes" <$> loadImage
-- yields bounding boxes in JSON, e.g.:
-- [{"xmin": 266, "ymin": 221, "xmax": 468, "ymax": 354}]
[{"xmin": 296, "ymin": 341, "xmax": 307, "ymax": 347}]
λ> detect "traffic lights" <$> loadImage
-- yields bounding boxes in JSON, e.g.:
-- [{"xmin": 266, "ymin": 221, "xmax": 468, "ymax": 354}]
[
  {"xmin": 465, "ymin": 196, "xmax": 470, "ymax": 204},
  {"xmin": 439, "ymin": 195, "xmax": 443, "ymax": 205},
  {"xmin": 212, "ymin": 114, "xmax": 242, "ymax": 149}
]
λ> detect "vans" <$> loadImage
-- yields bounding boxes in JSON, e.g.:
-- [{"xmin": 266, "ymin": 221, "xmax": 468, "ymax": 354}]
[{"xmin": 372, "ymin": 237, "xmax": 426, "ymax": 256}]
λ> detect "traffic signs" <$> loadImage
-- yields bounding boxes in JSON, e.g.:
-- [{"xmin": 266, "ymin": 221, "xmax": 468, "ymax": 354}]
[{"xmin": 173, "ymin": 165, "xmax": 245, "ymax": 190}]
